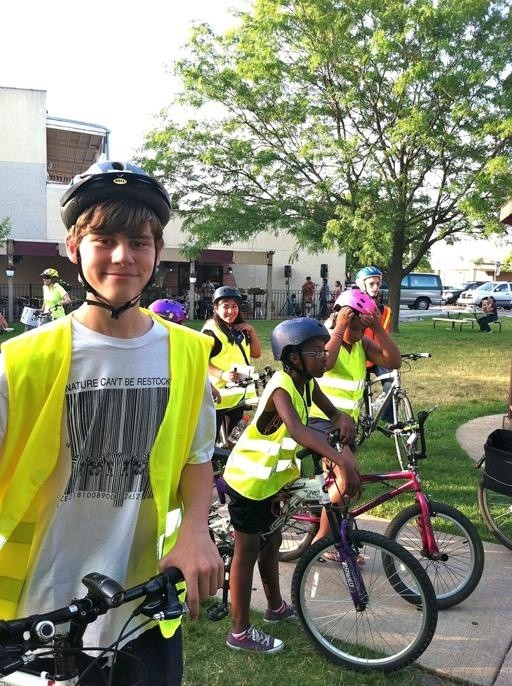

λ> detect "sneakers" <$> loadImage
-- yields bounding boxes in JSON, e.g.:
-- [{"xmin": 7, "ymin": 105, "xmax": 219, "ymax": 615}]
[
  {"xmin": 225, "ymin": 624, "xmax": 284, "ymax": 654},
  {"xmin": 263, "ymin": 602, "xmax": 295, "ymax": 624},
  {"xmin": 311, "ymin": 540, "xmax": 343, "ymax": 564}
]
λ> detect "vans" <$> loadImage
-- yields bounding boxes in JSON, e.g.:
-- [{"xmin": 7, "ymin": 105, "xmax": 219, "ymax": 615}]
[{"xmin": 380, "ymin": 272, "xmax": 442, "ymax": 310}]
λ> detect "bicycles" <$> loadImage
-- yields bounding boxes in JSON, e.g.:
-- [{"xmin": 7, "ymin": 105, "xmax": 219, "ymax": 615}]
[
  {"xmin": 475, "ymin": 407, "xmax": 512, "ymax": 555},
  {"xmin": 0, "ymin": 295, "xmax": 83, "ymax": 322},
  {"xmin": 32, "ymin": 303, "xmax": 61, "ymax": 327},
  {"xmin": 0, "ymin": 567, "xmax": 189, "ymax": 686}
]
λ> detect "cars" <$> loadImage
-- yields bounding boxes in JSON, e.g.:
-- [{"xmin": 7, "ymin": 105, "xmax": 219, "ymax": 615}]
[{"xmin": 442, "ymin": 280, "xmax": 512, "ymax": 309}]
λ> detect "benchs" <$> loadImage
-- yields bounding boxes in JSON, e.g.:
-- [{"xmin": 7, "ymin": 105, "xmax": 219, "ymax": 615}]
[
  {"xmin": 463, "ymin": 318, "xmax": 502, "ymax": 332},
  {"xmin": 432, "ymin": 318, "xmax": 471, "ymax": 332}
]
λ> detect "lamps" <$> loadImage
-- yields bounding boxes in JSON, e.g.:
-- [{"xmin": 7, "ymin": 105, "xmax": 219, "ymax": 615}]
[{"xmin": 228, "ymin": 267, "xmax": 233, "ymax": 274}]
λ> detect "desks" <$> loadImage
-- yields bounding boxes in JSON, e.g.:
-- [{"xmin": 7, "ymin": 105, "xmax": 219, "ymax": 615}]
[{"xmin": 442, "ymin": 308, "xmax": 485, "ymax": 331}]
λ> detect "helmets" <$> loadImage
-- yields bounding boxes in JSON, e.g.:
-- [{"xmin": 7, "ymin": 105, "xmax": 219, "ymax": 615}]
[
  {"xmin": 149, "ymin": 299, "xmax": 187, "ymax": 323},
  {"xmin": 332, "ymin": 289, "xmax": 381, "ymax": 320},
  {"xmin": 211, "ymin": 286, "xmax": 242, "ymax": 303},
  {"xmin": 271, "ymin": 316, "xmax": 331, "ymax": 360},
  {"xmin": 40, "ymin": 268, "xmax": 60, "ymax": 279},
  {"xmin": 355, "ymin": 267, "xmax": 383, "ymax": 289},
  {"xmin": 59, "ymin": 161, "xmax": 171, "ymax": 229}
]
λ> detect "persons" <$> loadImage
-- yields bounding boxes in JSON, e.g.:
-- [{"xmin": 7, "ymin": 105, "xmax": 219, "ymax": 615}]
[
  {"xmin": 330, "ymin": 280, "xmax": 342, "ymax": 305},
  {"xmin": 222, "ymin": 317, "xmax": 363, "ymax": 652},
  {"xmin": 147, "ymin": 298, "xmax": 223, "ymax": 404},
  {"xmin": 1, "ymin": 310, "xmax": 9, "ymax": 331},
  {"xmin": 1, "ymin": 160, "xmax": 225, "ymax": 686},
  {"xmin": 199, "ymin": 277, "xmax": 215, "ymax": 319},
  {"xmin": 319, "ymin": 278, "xmax": 329, "ymax": 312},
  {"xmin": 302, "ymin": 276, "xmax": 316, "ymax": 317},
  {"xmin": 200, "ymin": 285, "xmax": 262, "ymax": 482},
  {"xmin": 39, "ymin": 268, "xmax": 72, "ymax": 320},
  {"xmin": 477, "ymin": 296, "xmax": 498, "ymax": 334},
  {"xmin": 198, "ymin": 286, "xmax": 214, "ymax": 319},
  {"xmin": 305, "ymin": 288, "xmax": 402, "ymax": 566},
  {"xmin": 355, "ymin": 266, "xmax": 395, "ymax": 424}
]
